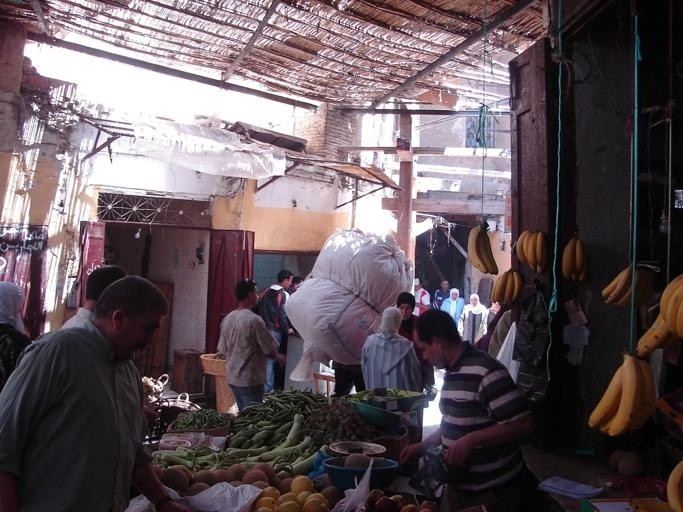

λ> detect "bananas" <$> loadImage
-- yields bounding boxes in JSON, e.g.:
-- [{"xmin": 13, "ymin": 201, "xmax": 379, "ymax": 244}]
[
  {"xmin": 562, "ymin": 239, "xmax": 586, "ymax": 281},
  {"xmin": 588, "ymin": 266, "xmax": 683, "ymax": 435},
  {"xmin": 468, "ymin": 221, "xmax": 498, "ymax": 275},
  {"xmin": 667, "ymin": 460, "xmax": 683, "ymax": 512},
  {"xmin": 516, "ymin": 231, "xmax": 546, "ymax": 273},
  {"xmin": 492, "ymin": 270, "xmax": 522, "ymax": 303}
]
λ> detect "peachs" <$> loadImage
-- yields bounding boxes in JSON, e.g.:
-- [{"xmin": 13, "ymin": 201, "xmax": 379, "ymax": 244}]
[{"xmin": 154, "ymin": 453, "xmax": 369, "ymax": 508}]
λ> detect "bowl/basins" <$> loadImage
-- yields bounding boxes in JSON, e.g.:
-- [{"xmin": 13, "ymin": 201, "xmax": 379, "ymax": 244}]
[
  {"xmin": 322, "ymin": 456, "xmax": 398, "ymax": 490},
  {"xmin": 326, "ymin": 441, "xmax": 386, "ymax": 454}
]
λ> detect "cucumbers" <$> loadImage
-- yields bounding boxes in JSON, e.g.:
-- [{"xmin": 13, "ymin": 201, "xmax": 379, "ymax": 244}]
[{"xmin": 229, "ymin": 390, "xmax": 328, "ymax": 449}]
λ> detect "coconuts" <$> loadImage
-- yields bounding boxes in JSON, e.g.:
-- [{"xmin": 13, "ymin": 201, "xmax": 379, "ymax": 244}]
[
  {"xmin": 610, "ymin": 450, "xmax": 624, "ymax": 468},
  {"xmin": 618, "ymin": 453, "xmax": 641, "ymax": 477}
]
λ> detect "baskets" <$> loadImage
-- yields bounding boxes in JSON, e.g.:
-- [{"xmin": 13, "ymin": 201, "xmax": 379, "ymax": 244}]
[{"xmin": 201, "ymin": 354, "xmax": 225, "ymax": 376}]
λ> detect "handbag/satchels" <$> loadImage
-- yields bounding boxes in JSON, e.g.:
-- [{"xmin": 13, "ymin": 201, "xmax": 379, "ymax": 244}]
[{"xmin": 423, "ymin": 446, "xmax": 474, "ymax": 483}]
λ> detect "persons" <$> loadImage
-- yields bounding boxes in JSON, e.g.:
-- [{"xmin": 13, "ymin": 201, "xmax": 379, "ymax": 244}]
[
  {"xmin": 258, "ymin": 270, "xmax": 294, "ymax": 392},
  {"xmin": 359, "ymin": 305, "xmax": 419, "ymax": 438},
  {"xmin": 0, "ymin": 281, "xmax": 35, "ymax": 388},
  {"xmin": 59, "ymin": 267, "xmax": 127, "ymax": 332},
  {"xmin": 412, "ymin": 278, "xmax": 499, "ymax": 347},
  {"xmin": 0, "ymin": 274, "xmax": 198, "ymax": 511},
  {"xmin": 216, "ymin": 278, "xmax": 286, "ymax": 406},
  {"xmin": 281, "ymin": 277, "xmax": 303, "ymax": 296},
  {"xmin": 325, "ymin": 361, "xmax": 369, "ymax": 396},
  {"xmin": 396, "ymin": 311, "xmax": 541, "ymax": 511},
  {"xmin": 392, "ymin": 295, "xmax": 435, "ymax": 407}
]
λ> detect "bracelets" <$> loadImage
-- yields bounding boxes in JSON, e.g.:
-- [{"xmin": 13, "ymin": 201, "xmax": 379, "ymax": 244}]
[{"xmin": 151, "ymin": 496, "xmax": 173, "ymax": 506}]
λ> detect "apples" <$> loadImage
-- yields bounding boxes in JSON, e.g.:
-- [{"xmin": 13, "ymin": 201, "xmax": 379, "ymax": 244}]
[{"xmin": 254, "ymin": 475, "xmax": 436, "ymax": 512}]
